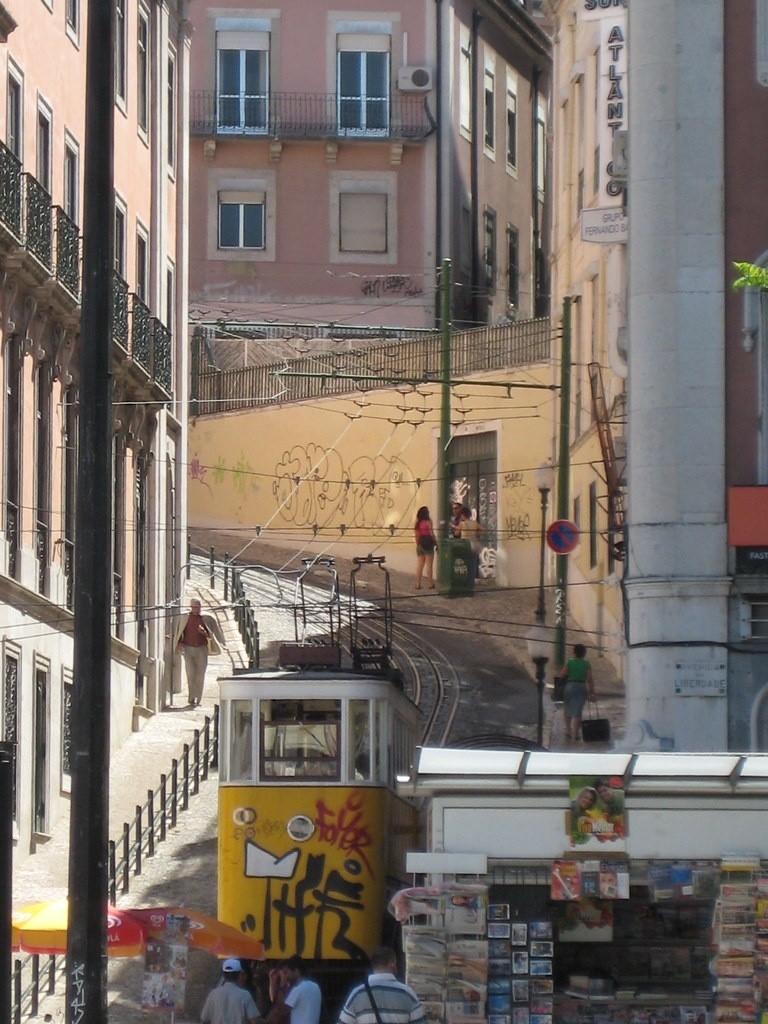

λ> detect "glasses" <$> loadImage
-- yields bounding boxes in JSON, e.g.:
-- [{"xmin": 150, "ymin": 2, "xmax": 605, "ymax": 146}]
[{"xmin": 452, "ymin": 506, "xmax": 459, "ymax": 510}]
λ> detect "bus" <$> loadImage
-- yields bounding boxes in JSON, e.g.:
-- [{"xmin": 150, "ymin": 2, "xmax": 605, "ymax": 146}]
[{"xmin": 217, "ymin": 553, "xmax": 421, "ymax": 961}]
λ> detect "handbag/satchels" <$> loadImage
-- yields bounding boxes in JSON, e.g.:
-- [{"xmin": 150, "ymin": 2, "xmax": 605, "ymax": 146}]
[
  {"xmin": 551, "ymin": 675, "xmax": 568, "ymax": 701},
  {"xmin": 419, "ymin": 535, "xmax": 434, "ymax": 549}
]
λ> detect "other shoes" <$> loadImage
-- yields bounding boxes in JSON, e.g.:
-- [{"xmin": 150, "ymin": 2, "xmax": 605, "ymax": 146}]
[{"xmin": 190, "ymin": 700, "xmax": 200, "ymax": 706}]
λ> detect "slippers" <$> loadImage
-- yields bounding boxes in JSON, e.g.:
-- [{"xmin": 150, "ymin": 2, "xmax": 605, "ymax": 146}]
[
  {"xmin": 416, "ymin": 586, "xmax": 419, "ymax": 589},
  {"xmin": 428, "ymin": 584, "xmax": 435, "ymax": 588}
]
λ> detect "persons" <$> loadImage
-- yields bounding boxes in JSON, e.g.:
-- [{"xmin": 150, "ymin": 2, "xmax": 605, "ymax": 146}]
[
  {"xmin": 448, "ymin": 502, "xmax": 486, "ymax": 579},
  {"xmin": 571, "ymin": 779, "xmax": 625, "ymax": 827},
  {"xmin": 199, "ymin": 954, "xmax": 321, "ymax": 1024},
  {"xmin": 173, "ymin": 599, "xmax": 228, "ymax": 706},
  {"xmin": 414, "ymin": 506, "xmax": 439, "ymax": 589},
  {"xmin": 451, "ymin": 896, "xmax": 478, "ymax": 913},
  {"xmin": 354, "ymin": 712, "xmax": 379, "ymax": 782},
  {"xmin": 337, "ymin": 949, "xmax": 425, "ymax": 1024},
  {"xmin": 559, "ymin": 643, "xmax": 593, "ymax": 739}
]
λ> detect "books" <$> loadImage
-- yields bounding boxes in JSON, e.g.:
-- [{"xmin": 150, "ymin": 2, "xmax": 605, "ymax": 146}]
[{"xmin": 615, "ymin": 984, "xmax": 715, "ymax": 1000}]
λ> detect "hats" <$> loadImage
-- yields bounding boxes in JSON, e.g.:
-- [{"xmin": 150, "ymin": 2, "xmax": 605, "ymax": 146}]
[{"xmin": 222, "ymin": 959, "xmax": 241, "ymax": 972}]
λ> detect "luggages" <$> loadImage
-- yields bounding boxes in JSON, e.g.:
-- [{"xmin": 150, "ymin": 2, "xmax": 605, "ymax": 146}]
[{"xmin": 580, "ymin": 700, "xmax": 611, "ymax": 743}]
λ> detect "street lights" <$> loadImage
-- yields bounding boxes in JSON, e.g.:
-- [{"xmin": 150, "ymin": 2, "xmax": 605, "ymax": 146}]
[
  {"xmin": 527, "ymin": 622, "xmax": 552, "ymax": 745},
  {"xmin": 533, "ymin": 462, "xmax": 554, "ymax": 625}
]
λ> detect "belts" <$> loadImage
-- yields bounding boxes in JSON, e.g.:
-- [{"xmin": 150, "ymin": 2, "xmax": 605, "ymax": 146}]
[{"xmin": 184, "ymin": 643, "xmax": 207, "ymax": 647}]
